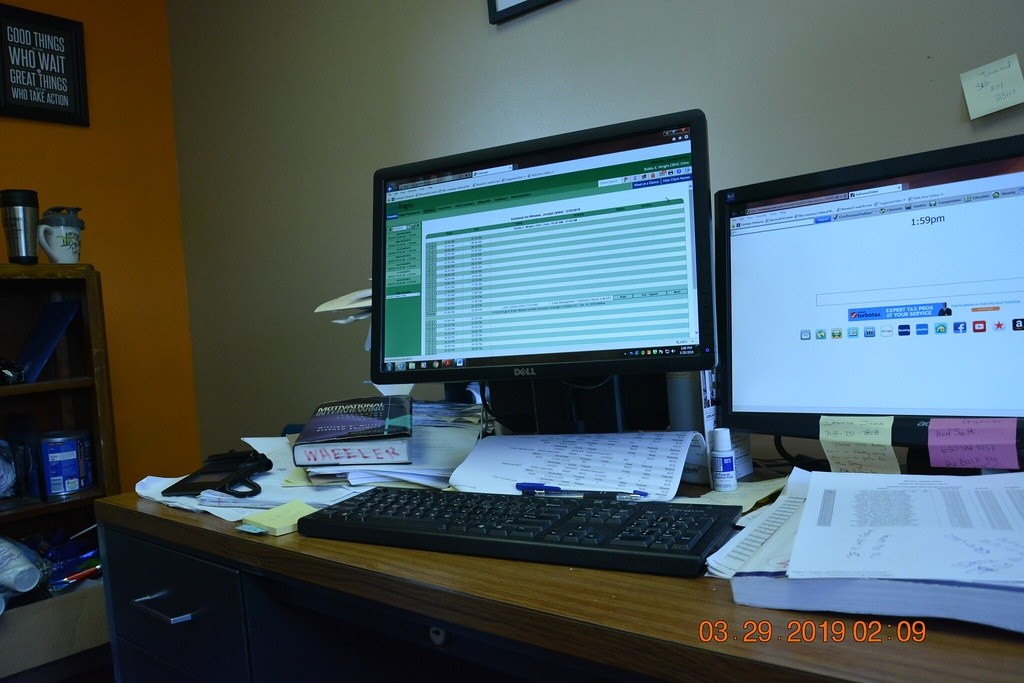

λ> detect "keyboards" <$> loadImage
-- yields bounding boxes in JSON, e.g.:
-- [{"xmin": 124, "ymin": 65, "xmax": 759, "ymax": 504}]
[{"xmin": 298, "ymin": 486, "xmax": 744, "ymax": 579}]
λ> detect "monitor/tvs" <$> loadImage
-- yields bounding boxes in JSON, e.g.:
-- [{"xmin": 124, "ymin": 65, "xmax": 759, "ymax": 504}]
[
  {"xmin": 370, "ymin": 108, "xmax": 719, "ymax": 436},
  {"xmin": 715, "ymin": 133, "xmax": 1024, "ymax": 476}
]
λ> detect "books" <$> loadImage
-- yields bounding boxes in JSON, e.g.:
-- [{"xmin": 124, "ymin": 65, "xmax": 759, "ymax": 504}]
[
  {"xmin": 293, "ymin": 393, "xmax": 488, "ymax": 466},
  {"xmin": 731, "ymin": 465, "xmax": 1024, "ymax": 633}
]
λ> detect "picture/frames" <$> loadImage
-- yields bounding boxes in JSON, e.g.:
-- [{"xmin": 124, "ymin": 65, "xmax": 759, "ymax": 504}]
[
  {"xmin": 0, "ymin": 3, "xmax": 90, "ymax": 126},
  {"xmin": 488, "ymin": 0, "xmax": 562, "ymax": 25}
]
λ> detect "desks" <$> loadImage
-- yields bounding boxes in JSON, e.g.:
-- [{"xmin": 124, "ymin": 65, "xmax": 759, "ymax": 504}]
[{"xmin": 94, "ymin": 493, "xmax": 1024, "ymax": 683}]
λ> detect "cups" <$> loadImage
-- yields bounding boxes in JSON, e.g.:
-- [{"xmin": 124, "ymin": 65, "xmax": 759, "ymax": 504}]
[
  {"xmin": 0, "ymin": 189, "xmax": 40, "ymax": 263},
  {"xmin": 667, "ymin": 371, "xmax": 706, "ymax": 440},
  {"xmin": 0, "ymin": 537, "xmax": 41, "ymax": 615},
  {"xmin": 39, "ymin": 205, "xmax": 85, "ymax": 264}
]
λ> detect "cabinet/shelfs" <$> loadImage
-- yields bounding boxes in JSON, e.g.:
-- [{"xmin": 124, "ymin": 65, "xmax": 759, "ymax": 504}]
[{"xmin": 0, "ymin": 264, "xmax": 121, "ymax": 681}]
